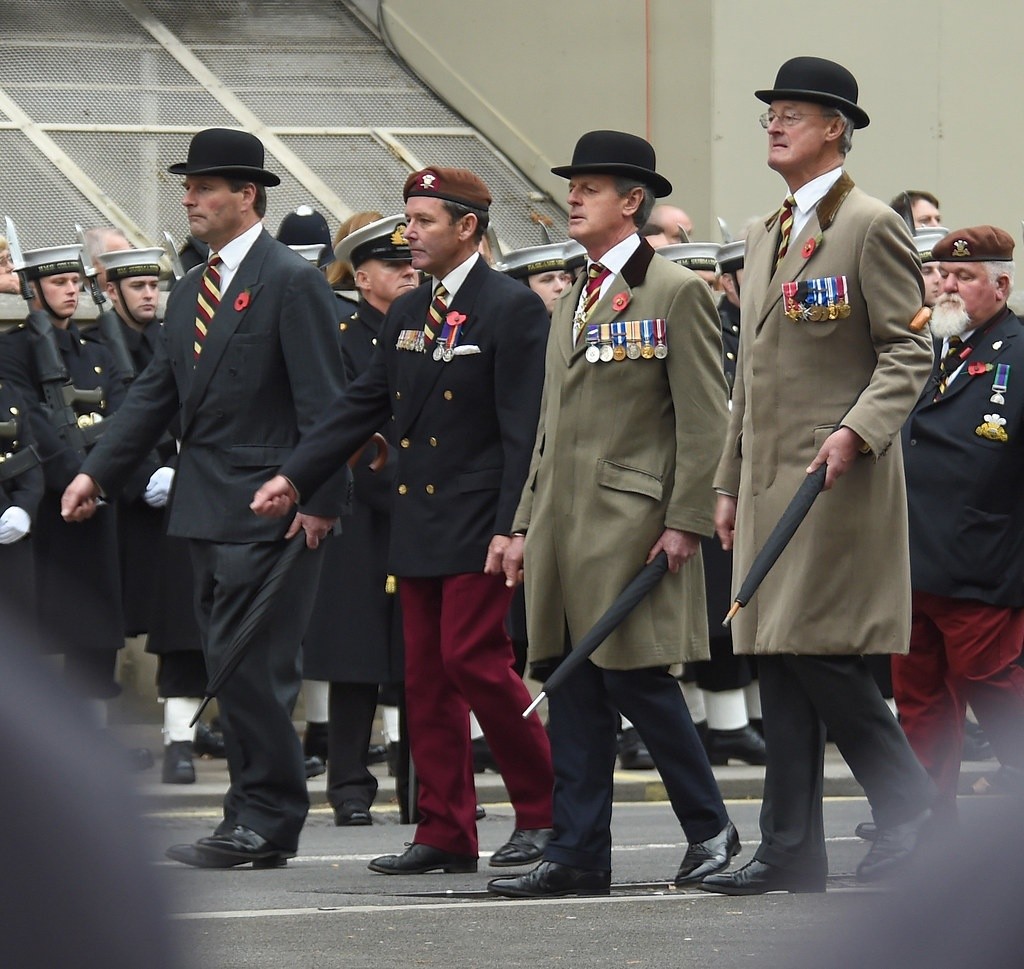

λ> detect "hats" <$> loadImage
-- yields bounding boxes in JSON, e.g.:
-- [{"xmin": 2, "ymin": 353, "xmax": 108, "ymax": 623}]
[
  {"xmin": 23, "ymin": 243, "xmax": 85, "ymax": 281},
  {"xmin": 931, "ymin": 225, "xmax": 1015, "ymax": 263},
  {"xmin": 913, "ymin": 234, "xmax": 944, "ymax": 263},
  {"xmin": 166, "ymin": 129, "xmax": 280, "ymax": 194},
  {"xmin": 96, "ymin": 247, "xmax": 167, "ymax": 280},
  {"xmin": 717, "ymin": 241, "xmax": 746, "ymax": 273},
  {"xmin": 755, "ymin": 56, "xmax": 870, "ymax": 131},
  {"xmin": 563, "ymin": 240, "xmax": 590, "ymax": 274},
  {"xmin": 403, "ymin": 166, "xmax": 493, "ymax": 212},
  {"xmin": 551, "ymin": 130, "xmax": 672, "ymax": 202},
  {"xmin": 333, "ymin": 214, "xmax": 413, "ymax": 271},
  {"xmin": 654, "ymin": 243, "xmax": 720, "ymax": 271},
  {"xmin": 500, "ymin": 242, "xmax": 565, "ymax": 279},
  {"xmin": 916, "ymin": 226, "xmax": 950, "ymax": 236}
]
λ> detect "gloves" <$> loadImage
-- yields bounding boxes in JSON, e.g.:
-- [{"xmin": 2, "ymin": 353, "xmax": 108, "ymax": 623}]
[
  {"xmin": 145, "ymin": 467, "xmax": 175, "ymax": 507},
  {"xmin": 0, "ymin": 507, "xmax": 31, "ymax": 545}
]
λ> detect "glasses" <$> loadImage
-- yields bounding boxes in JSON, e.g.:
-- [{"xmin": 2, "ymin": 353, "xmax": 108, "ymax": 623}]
[
  {"xmin": 758, "ymin": 112, "xmax": 838, "ymax": 128},
  {"xmin": 0, "ymin": 254, "xmax": 13, "ymax": 267}
]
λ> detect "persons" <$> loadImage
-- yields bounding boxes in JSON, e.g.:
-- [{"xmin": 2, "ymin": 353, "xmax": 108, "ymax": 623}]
[
  {"xmin": 63, "ymin": 127, "xmax": 353, "ymax": 871},
  {"xmin": 0, "ymin": 58, "xmax": 1024, "ymax": 969},
  {"xmin": 248, "ymin": 165, "xmax": 552, "ymax": 874},
  {"xmin": 484, "ymin": 129, "xmax": 742, "ymax": 901}
]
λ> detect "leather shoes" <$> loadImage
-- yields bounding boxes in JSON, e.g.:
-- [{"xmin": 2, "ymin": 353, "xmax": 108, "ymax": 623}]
[
  {"xmin": 167, "ymin": 825, "xmax": 297, "ymax": 871},
  {"xmin": 487, "ymin": 862, "xmax": 611, "ymax": 897},
  {"xmin": 673, "ymin": 820, "xmax": 741, "ymax": 888},
  {"xmin": 490, "ymin": 828, "xmax": 552, "ymax": 867},
  {"xmin": 702, "ymin": 859, "xmax": 828, "ymax": 894},
  {"xmin": 369, "ymin": 843, "xmax": 477, "ymax": 874},
  {"xmin": 857, "ymin": 809, "xmax": 932, "ymax": 882}
]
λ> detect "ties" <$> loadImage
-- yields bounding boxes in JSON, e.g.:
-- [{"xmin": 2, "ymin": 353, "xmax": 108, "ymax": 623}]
[
  {"xmin": 192, "ymin": 253, "xmax": 223, "ymax": 369},
  {"xmin": 774, "ymin": 196, "xmax": 796, "ymax": 272},
  {"xmin": 934, "ymin": 336, "xmax": 960, "ymax": 402},
  {"xmin": 576, "ymin": 263, "xmax": 612, "ymax": 342},
  {"xmin": 422, "ymin": 282, "xmax": 448, "ymax": 355}
]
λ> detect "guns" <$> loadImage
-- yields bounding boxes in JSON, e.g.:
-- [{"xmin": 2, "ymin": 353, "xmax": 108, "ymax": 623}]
[
  {"xmin": 75, "ymin": 224, "xmax": 135, "ymax": 392},
  {"xmin": 0, "ymin": 417, "xmax": 44, "ymax": 519},
  {"xmin": 5, "ymin": 215, "xmax": 117, "ymax": 507}
]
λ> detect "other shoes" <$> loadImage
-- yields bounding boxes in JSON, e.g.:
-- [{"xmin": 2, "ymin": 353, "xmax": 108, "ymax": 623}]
[
  {"xmin": 335, "ymin": 804, "xmax": 372, "ymax": 825},
  {"xmin": 708, "ymin": 726, "xmax": 769, "ymax": 765},
  {"xmin": 303, "ymin": 754, "xmax": 325, "ymax": 778},
  {"xmin": 475, "ymin": 804, "xmax": 486, "ymax": 820},
  {"xmin": 163, "ymin": 741, "xmax": 194, "ymax": 784},
  {"xmin": 194, "ymin": 722, "xmax": 225, "ymax": 758},
  {"xmin": 617, "ymin": 729, "xmax": 655, "ymax": 769},
  {"xmin": 965, "ymin": 721, "xmax": 992, "ymax": 761},
  {"xmin": 855, "ymin": 822, "xmax": 877, "ymax": 840},
  {"xmin": 302, "ymin": 742, "xmax": 386, "ymax": 765},
  {"xmin": 86, "ymin": 730, "xmax": 152, "ymax": 773}
]
